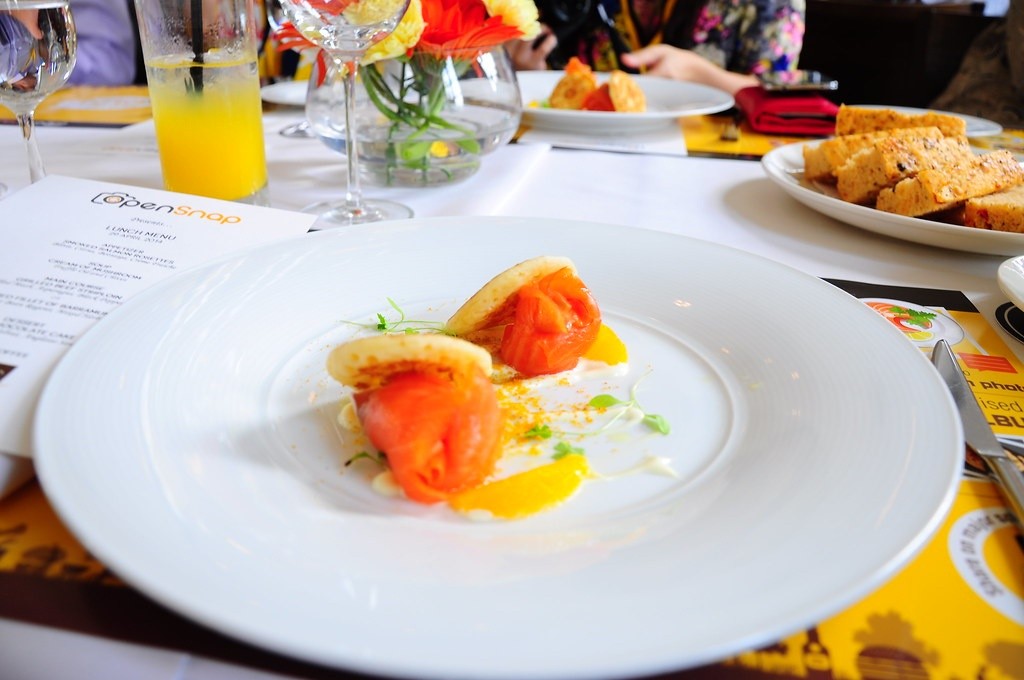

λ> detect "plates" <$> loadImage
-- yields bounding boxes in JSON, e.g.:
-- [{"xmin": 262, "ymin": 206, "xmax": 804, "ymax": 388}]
[
  {"xmin": 454, "ymin": 69, "xmax": 736, "ymax": 136},
  {"xmin": 29, "ymin": 215, "xmax": 967, "ymax": 680},
  {"xmin": 761, "ymin": 139, "xmax": 1024, "ymax": 255},
  {"xmin": 997, "ymin": 256, "xmax": 1024, "ymax": 312},
  {"xmin": 848, "ymin": 105, "xmax": 1004, "ymax": 137}
]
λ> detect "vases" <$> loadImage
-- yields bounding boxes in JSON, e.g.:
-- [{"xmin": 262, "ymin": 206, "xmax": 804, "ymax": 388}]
[{"xmin": 302, "ymin": 44, "xmax": 522, "ymax": 186}]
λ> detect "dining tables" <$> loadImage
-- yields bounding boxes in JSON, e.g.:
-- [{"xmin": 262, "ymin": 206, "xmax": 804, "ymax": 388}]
[{"xmin": 0, "ymin": 89, "xmax": 1024, "ymax": 680}]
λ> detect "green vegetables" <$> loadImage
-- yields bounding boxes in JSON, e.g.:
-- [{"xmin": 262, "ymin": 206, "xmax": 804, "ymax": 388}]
[{"xmin": 334, "ymin": 297, "xmax": 669, "ymax": 468}]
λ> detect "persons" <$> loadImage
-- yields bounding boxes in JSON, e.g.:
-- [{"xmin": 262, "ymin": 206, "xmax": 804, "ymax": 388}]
[
  {"xmin": 160, "ymin": 0, "xmax": 318, "ymax": 92},
  {"xmin": 500, "ymin": 0, "xmax": 805, "ymax": 105},
  {"xmin": 0, "ymin": 0, "xmax": 135, "ymax": 91}
]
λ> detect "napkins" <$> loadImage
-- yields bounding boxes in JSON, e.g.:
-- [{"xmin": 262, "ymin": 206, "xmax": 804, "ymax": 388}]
[{"xmin": 736, "ymin": 85, "xmax": 838, "ymax": 135}]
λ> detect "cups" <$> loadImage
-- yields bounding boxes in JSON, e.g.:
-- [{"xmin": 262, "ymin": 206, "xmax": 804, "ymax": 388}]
[{"xmin": 133, "ymin": 0, "xmax": 276, "ymax": 198}]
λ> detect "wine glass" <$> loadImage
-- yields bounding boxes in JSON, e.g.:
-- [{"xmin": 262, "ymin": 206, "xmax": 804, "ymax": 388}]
[
  {"xmin": 294, "ymin": 0, "xmax": 417, "ymax": 233},
  {"xmin": 266, "ymin": 0, "xmax": 326, "ymax": 139},
  {"xmin": 0, "ymin": 0, "xmax": 78, "ymax": 185}
]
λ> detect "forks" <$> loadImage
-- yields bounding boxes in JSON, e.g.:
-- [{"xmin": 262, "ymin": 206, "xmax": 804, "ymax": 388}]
[{"xmin": 720, "ymin": 117, "xmax": 743, "ymax": 140}]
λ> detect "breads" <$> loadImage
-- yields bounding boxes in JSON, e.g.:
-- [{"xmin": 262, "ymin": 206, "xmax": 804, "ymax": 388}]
[{"xmin": 803, "ymin": 104, "xmax": 1024, "ymax": 234}]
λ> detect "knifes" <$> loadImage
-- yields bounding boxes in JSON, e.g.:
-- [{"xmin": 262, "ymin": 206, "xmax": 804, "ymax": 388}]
[{"xmin": 932, "ymin": 338, "xmax": 1024, "ymax": 527}]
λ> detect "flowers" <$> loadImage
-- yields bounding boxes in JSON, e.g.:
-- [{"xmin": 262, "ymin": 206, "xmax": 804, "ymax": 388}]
[{"xmin": 267, "ymin": 0, "xmax": 538, "ymax": 90}]
